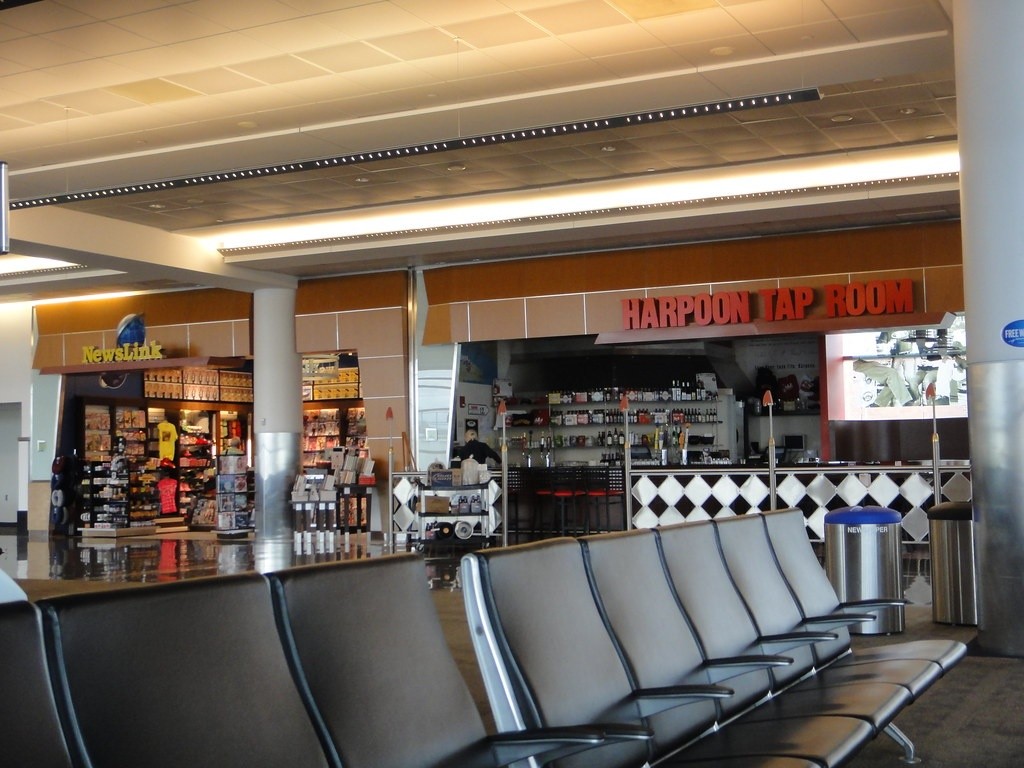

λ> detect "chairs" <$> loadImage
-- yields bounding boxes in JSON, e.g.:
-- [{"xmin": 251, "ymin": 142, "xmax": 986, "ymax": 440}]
[{"xmin": 0, "ymin": 507, "xmax": 967, "ymax": 768}]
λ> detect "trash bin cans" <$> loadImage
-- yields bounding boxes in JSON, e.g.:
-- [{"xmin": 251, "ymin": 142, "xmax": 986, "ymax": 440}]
[
  {"xmin": 824, "ymin": 506, "xmax": 905, "ymax": 634},
  {"xmin": 927, "ymin": 500, "xmax": 980, "ymax": 626}
]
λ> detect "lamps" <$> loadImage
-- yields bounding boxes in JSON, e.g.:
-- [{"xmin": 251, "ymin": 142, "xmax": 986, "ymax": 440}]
[
  {"xmin": 619, "ymin": 396, "xmax": 633, "ymax": 530},
  {"xmin": 498, "ymin": 400, "xmax": 508, "ymax": 547},
  {"xmin": 338, "ymin": 352, "xmax": 361, "ymax": 372},
  {"xmin": 763, "ymin": 390, "xmax": 777, "ymax": 511},
  {"xmin": 926, "ymin": 382, "xmax": 942, "ymax": 507}
]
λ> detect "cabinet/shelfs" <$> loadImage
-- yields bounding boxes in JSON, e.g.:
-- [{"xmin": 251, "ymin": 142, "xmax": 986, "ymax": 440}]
[
  {"xmin": 507, "ymin": 388, "xmax": 745, "ymax": 466},
  {"xmin": 418, "ymin": 477, "xmax": 503, "ymax": 556}
]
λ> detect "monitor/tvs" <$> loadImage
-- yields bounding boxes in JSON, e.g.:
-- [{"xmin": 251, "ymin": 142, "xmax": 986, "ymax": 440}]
[{"xmin": 784, "ymin": 433, "xmax": 806, "ymax": 451}]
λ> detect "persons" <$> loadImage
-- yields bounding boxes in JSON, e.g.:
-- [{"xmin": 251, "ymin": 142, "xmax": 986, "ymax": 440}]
[
  {"xmin": 458, "ymin": 430, "xmax": 501, "ymax": 469},
  {"xmin": 235, "ymin": 458, "xmax": 247, "ymax": 473},
  {"xmin": 850, "ymin": 332, "xmax": 966, "ymax": 407}
]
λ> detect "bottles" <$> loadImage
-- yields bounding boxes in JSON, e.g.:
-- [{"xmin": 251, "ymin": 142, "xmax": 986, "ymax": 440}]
[{"xmin": 491, "ymin": 379, "xmax": 738, "ymax": 470}]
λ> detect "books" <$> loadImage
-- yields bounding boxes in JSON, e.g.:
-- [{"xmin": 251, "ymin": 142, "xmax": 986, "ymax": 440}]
[{"xmin": 216, "ymin": 455, "xmax": 249, "ymax": 531}]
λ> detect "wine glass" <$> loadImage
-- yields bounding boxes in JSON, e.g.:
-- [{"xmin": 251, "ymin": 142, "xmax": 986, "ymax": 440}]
[{"xmin": 143, "ymin": 367, "xmax": 258, "ymax": 404}]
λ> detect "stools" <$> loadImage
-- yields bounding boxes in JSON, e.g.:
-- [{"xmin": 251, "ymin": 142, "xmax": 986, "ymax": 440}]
[
  {"xmin": 557, "ymin": 489, "xmax": 584, "ymax": 536},
  {"xmin": 508, "ymin": 486, "xmax": 525, "ymax": 545},
  {"xmin": 533, "ymin": 488, "xmax": 565, "ymax": 540},
  {"xmin": 589, "ymin": 489, "xmax": 625, "ymax": 536}
]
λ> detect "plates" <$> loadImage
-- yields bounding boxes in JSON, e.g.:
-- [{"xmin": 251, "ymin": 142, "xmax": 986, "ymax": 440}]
[{"xmin": 434, "ymin": 521, "xmax": 474, "ymax": 540}]
